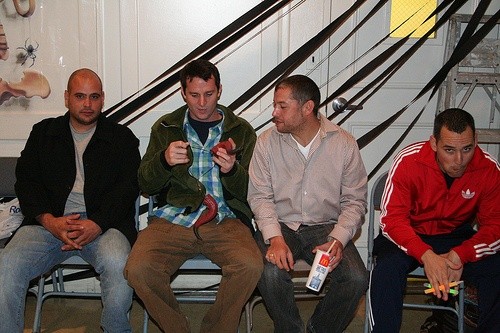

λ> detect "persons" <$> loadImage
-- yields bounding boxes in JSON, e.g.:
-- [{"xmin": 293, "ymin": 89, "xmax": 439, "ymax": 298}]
[
  {"xmin": 0, "ymin": 68, "xmax": 141, "ymax": 333},
  {"xmin": 369, "ymin": 201, "xmax": 369, "ymax": 202},
  {"xmin": 123, "ymin": 60, "xmax": 263, "ymax": 333},
  {"xmin": 367, "ymin": 108, "xmax": 500, "ymax": 333},
  {"xmin": 247, "ymin": 74, "xmax": 370, "ymax": 333}
]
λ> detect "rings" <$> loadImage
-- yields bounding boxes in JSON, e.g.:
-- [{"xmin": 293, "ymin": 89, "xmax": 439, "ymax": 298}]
[{"xmin": 268, "ymin": 252, "xmax": 274, "ymax": 256}]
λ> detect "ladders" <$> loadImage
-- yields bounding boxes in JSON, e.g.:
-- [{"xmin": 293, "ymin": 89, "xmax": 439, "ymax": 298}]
[{"xmin": 435, "ymin": 14, "xmax": 500, "ymax": 166}]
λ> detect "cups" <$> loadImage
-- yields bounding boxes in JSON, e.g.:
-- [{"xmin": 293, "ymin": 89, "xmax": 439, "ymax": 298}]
[{"xmin": 306, "ymin": 248, "xmax": 336, "ymax": 292}]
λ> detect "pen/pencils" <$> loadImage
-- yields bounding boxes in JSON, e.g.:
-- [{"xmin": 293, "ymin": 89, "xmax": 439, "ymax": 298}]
[{"xmin": 424, "ymin": 280, "xmax": 464, "ymax": 295}]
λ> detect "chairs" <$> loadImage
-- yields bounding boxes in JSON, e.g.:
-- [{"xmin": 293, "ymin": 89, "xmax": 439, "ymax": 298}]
[
  {"xmin": 28, "ymin": 256, "xmax": 102, "ymax": 333},
  {"xmin": 363, "ymin": 171, "xmax": 465, "ymax": 333},
  {"xmin": 244, "ymin": 263, "xmax": 332, "ymax": 333},
  {"xmin": 142, "ymin": 193, "xmax": 222, "ymax": 333}
]
